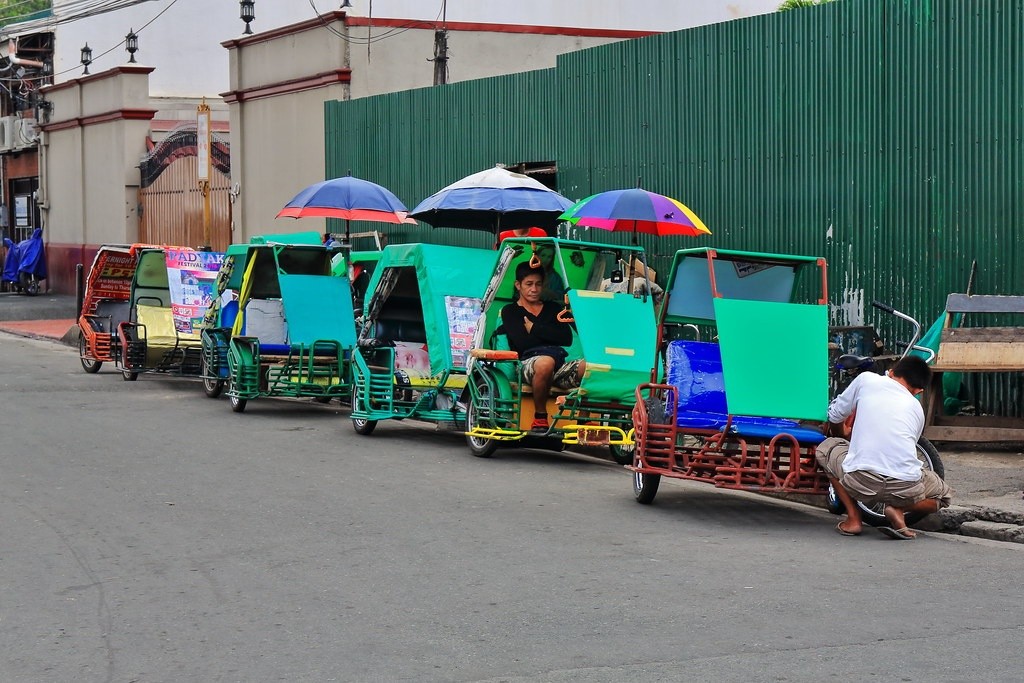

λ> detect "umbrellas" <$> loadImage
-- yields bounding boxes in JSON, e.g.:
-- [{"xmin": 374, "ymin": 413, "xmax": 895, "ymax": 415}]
[
  {"xmin": 558, "ymin": 175, "xmax": 713, "ymax": 293},
  {"xmin": 275, "ymin": 170, "xmax": 419, "ymax": 279},
  {"xmin": 406, "ymin": 165, "xmax": 577, "ymax": 252}
]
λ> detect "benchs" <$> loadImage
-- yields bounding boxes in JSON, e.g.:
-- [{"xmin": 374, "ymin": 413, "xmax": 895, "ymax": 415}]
[
  {"xmin": 96, "ymin": 298, "xmax": 130, "ymax": 332},
  {"xmin": 135, "ymin": 304, "xmax": 205, "ymax": 349},
  {"xmin": 369, "ymin": 294, "xmax": 431, "ymax": 374},
  {"xmin": 222, "ymin": 300, "xmax": 345, "ymax": 357},
  {"xmin": 665, "ymin": 340, "xmax": 802, "ymax": 430},
  {"xmin": 496, "ymin": 307, "xmax": 583, "ymax": 395}
]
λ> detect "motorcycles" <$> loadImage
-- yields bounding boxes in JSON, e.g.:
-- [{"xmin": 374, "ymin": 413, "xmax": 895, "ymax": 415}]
[{"xmin": 5, "ymin": 227, "xmax": 44, "ymax": 296}]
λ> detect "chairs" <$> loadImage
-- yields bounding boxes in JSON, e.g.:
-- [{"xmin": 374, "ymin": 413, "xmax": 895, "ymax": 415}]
[{"xmin": 720, "ymin": 424, "xmax": 825, "ymax": 444}]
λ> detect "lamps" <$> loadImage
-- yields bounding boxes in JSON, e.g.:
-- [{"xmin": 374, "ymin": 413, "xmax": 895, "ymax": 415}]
[
  {"xmin": 125, "ymin": 27, "xmax": 139, "ymax": 63},
  {"xmin": 79, "ymin": 43, "xmax": 93, "ymax": 74},
  {"xmin": 239, "ymin": 0, "xmax": 255, "ymax": 34},
  {"xmin": 42, "ymin": 53, "xmax": 52, "ymax": 84}
]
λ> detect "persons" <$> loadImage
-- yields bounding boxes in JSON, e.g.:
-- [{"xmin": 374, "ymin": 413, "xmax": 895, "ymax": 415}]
[
  {"xmin": 499, "ymin": 226, "xmax": 547, "ymax": 249},
  {"xmin": 502, "ymin": 261, "xmax": 586, "ymax": 432},
  {"xmin": 816, "ymin": 354, "xmax": 952, "ymax": 539}
]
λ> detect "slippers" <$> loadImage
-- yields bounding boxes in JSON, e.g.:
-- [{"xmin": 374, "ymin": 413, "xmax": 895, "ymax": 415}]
[
  {"xmin": 876, "ymin": 526, "xmax": 913, "ymax": 540},
  {"xmin": 835, "ymin": 521, "xmax": 855, "ymax": 536}
]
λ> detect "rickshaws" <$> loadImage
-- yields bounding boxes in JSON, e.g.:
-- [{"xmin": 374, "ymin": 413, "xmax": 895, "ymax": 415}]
[{"xmin": 79, "ymin": 228, "xmax": 945, "ymax": 530}]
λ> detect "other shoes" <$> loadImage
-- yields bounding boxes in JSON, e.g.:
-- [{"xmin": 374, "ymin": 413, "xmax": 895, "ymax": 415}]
[
  {"xmin": 531, "ymin": 413, "xmax": 550, "ymax": 433},
  {"xmin": 577, "ymin": 420, "xmax": 599, "ymax": 426}
]
